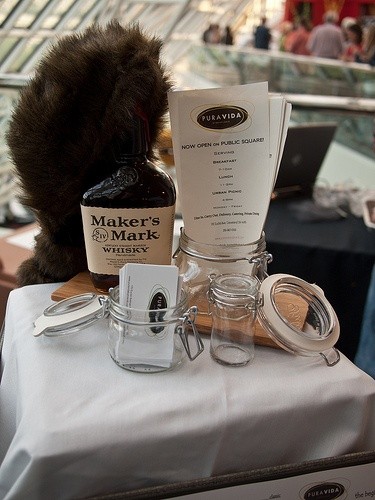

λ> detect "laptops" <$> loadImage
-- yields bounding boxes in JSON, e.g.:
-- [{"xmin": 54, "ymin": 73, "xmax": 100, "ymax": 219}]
[{"xmin": 271, "ymin": 122, "xmax": 337, "ymax": 200}]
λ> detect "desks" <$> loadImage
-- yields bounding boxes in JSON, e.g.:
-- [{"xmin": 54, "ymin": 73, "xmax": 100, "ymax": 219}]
[{"xmin": 0, "ymin": 282, "xmax": 375, "ymax": 499}]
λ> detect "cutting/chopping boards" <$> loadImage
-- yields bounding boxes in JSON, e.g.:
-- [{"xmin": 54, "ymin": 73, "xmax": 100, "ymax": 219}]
[{"xmin": 51, "ymin": 269, "xmax": 315, "ymax": 352}]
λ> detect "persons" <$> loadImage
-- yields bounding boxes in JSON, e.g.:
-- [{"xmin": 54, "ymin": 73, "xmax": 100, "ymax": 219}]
[{"xmin": 203, "ymin": 8, "xmax": 373, "ymax": 59}]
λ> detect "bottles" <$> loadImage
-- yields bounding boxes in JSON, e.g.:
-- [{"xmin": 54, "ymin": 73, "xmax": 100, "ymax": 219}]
[{"xmin": 81, "ymin": 118, "xmax": 176, "ymax": 293}]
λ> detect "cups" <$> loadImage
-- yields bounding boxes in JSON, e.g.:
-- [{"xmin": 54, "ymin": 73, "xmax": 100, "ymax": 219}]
[
  {"xmin": 178, "ymin": 227, "xmax": 266, "ymax": 314},
  {"xmin": 210, "ymin": 273, "xmax": 260, "ymax": 367},
  {"xmin": 108, "ymin": 286, "xmax": 188, "ymax": 373}
]
